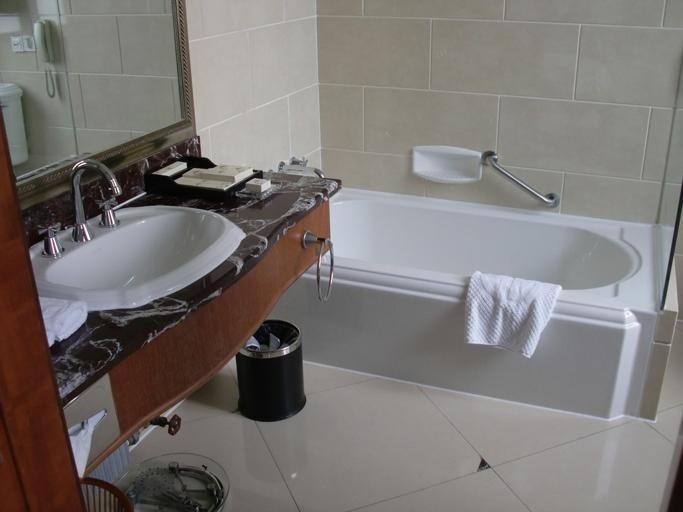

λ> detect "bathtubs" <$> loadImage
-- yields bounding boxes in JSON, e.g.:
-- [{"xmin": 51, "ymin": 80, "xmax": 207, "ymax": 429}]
[{"xmin": 272, "ymin": 180, "xmax": 660, "ymax": 422}]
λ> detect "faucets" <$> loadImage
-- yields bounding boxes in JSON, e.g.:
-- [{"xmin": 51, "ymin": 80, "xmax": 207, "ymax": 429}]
[{"xmin": 67, "ymin": 158, "xmax": 123, "ymax": 242}]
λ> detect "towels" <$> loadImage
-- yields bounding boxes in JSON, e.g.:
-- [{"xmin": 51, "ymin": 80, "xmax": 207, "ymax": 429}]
[
  {"xmin": 41, "ymin": 297, "xmax": 86, "ymax": 351},
  {"xmin": 461, "ymin": 269, "xmax": 562, "ymax": 361}
]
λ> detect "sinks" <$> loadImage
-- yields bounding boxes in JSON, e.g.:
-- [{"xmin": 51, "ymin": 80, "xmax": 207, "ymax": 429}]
[{"xmin": 29, "ymin": 206, "xmax": 246, "ymax": 309}]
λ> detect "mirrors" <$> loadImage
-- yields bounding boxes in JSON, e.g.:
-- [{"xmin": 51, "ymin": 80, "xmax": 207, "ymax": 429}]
[{"xmin": 0, "ymin": 1, "xmax": 196, "ymax": 202}]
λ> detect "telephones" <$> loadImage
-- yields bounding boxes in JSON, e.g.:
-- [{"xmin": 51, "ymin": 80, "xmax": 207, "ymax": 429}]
[{"xmin": 33, "ymin": 19, "xmax": 55, "ymax": 65}]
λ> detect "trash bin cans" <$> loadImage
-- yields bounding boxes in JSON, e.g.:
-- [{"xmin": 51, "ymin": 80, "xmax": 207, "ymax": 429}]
[{"xmin": 235, "ymin": 320, "xmax": 307, "ymax": 423}]
